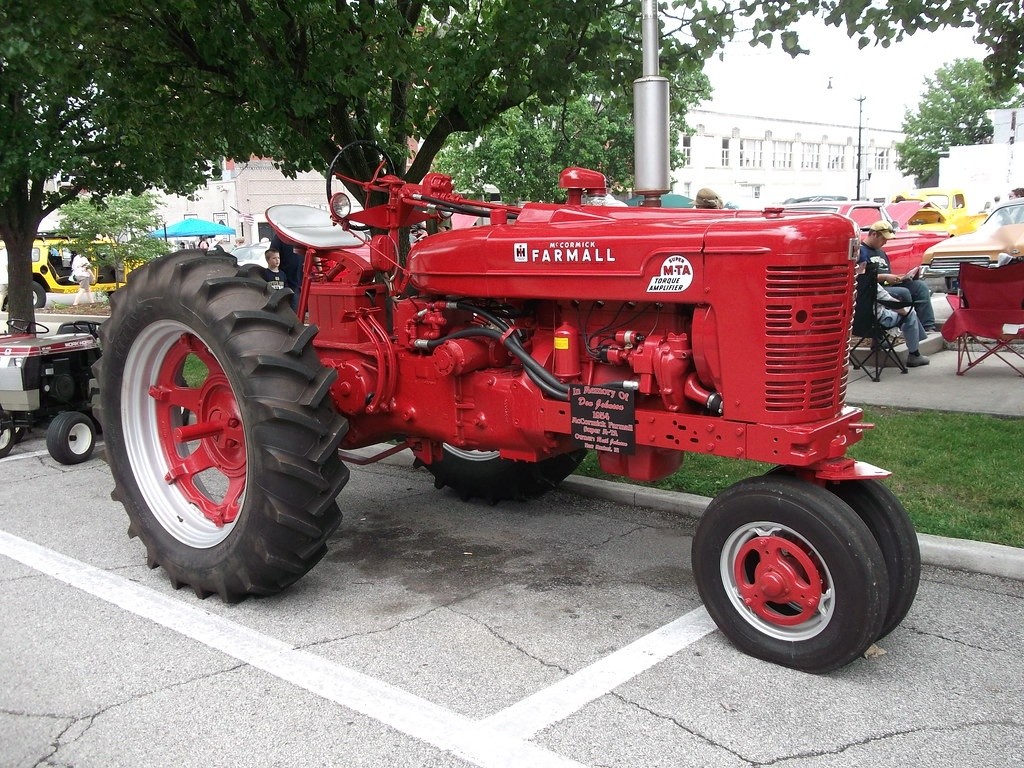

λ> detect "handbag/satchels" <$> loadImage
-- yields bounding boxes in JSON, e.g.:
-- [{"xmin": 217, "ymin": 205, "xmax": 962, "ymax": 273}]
[{"xmin": 68, "ymin": 271, "xmax": 79, "ymax": 283}]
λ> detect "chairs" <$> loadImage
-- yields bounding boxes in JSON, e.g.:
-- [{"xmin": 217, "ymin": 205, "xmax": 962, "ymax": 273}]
[
  {"xmin": 57, "ymin": 323, "xmax": 95, "ymax": 334},
  {"xmin": 265, "ymin": 204, "xmax": 366, "ymax": 328},
  {"xmin": 850, "ymin": 262, "xmax": 929, "ymax": 382},
  {"xmin": 941, "ymin": 253, "xmax": 1024, "ymax": 377}
]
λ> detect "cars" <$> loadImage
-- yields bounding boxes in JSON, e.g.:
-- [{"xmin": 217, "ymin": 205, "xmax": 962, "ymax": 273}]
[
  {"xmin": 228, "ymin": 240, "xmax": 271, "ymax": 270},
  {"xmin": 773, "ymin": 186, "xmax": 1024, "ymax": 298}
]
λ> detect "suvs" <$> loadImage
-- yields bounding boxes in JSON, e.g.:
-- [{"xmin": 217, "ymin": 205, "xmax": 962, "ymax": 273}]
[{"xmin": 0, "ymin": 231, "xmax": 142, "ymax": 313}]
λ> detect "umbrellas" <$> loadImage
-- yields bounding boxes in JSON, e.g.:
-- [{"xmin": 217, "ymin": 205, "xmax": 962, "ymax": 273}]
[{"xmin": 145, "ymin": 218, "xmax": 236, "ymax": 238}]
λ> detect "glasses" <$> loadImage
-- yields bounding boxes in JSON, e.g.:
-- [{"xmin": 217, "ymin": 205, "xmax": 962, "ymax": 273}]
[{"xmin": 876, "ymin": 227, "xmax": 895, "ymax": 234}]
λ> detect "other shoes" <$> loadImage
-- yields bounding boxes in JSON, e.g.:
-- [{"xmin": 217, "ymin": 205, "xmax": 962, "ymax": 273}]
[
  {"xmin": 906, "ymin": 353, "xmax": 930, "ymax": 367},
  {"xmin": 870, "ymin": 341, "xmax": 893, "ymax": 351}
]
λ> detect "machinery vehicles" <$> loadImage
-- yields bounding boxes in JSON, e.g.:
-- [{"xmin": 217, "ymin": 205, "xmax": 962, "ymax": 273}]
[{"xmin": 82, "ymin": 0, "xmax": 921, "ymax": 677}]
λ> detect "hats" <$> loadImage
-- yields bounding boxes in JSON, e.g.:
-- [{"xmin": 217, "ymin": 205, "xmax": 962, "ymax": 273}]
[
  {"xmin": 871, "ymin": 220, "xmax": 897, "ymax": 239},
  {"xmin": 688, "ymin": 187, "xmax": 719, "ymax": 207}
]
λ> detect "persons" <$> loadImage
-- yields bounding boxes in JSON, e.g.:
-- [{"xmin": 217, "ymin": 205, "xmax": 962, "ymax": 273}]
[
  {"xmin": 984, "ymin": 188, "xmax": 1024, "ymax": 212},
  {"xmin": 857, "ymin": 220, "xmax": 936, "ymax": 367},
  {"xmin": 72, "ymin": 248, "xmax": 97, "ymax": 308},
  {"xmin": 688, "ymin": 188, "xmax": 724, "ymax": 209},
  {"xmin": 229, "ymin": 234, "xmax": 305, "ymax": 314}
]
